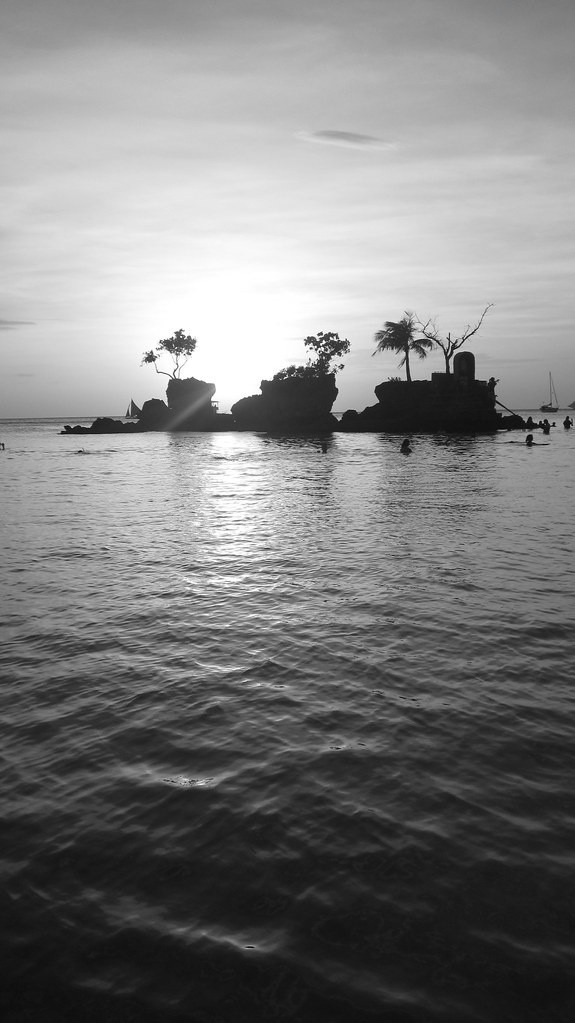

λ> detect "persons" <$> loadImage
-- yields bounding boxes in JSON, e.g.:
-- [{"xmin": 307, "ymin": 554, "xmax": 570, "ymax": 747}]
[
  {"xmin": 538, "ymin": 419, "xmax": 556, "ymax": 435},
  {"xmin": 526, "ymin": 434, "xmax": 536, "ymax": 445},
  {"xmin": 0, "ymin": 442, "xmax": 4, "ymax": 450},
  {"xmin": 563, "ymin": 416, "xmax": 573, "ymax": 429},
  {"xmin": 321, "ymin": 444, "xmax": 327, "ymax": 453},
  {"xmin": 400, "ymin": 438, "xmax": 412, "ymax": 452},
  {"xmin": 526, "ymin": 416, "xmax": 537, "ymax": 429}
]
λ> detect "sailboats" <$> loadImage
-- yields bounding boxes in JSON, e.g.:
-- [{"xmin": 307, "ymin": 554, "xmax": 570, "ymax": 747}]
[
  {"xmin": 541, "ymin": 371, "xmax": 559, "ymax": 412},
  {"xmin": 125, "ymin": 409, "xmax": 131, "ymax": 419},
  {"xmin": 131, "ymin": 399, "xmax": 142, "ymax": 418}
]
